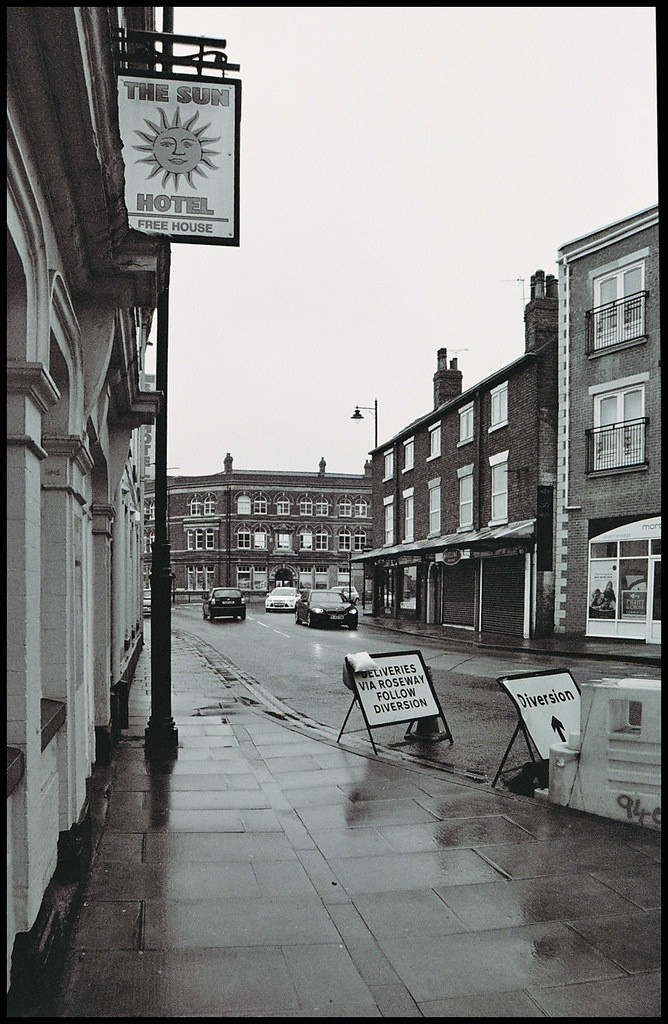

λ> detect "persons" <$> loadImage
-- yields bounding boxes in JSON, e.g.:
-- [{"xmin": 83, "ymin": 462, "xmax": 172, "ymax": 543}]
[{"xmin": 590, "ymin": 581, "xmax": 616, "ymax": 611}]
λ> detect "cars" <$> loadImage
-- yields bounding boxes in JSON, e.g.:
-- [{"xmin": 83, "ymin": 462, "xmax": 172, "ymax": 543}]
[
  {"xmin": 143, "ymin": 589, "xmax": 151, "ymax": 615},
  {"xmin": 265, "ymin": 587, "xmax": 302, "ymax": 612},
  {"xmin": 201, "ymin": 587, "xmax": 246, "ymax": 621},
  {"xmin": 295, "ymin": 586, "xmax": 361, "ymax": 630}
]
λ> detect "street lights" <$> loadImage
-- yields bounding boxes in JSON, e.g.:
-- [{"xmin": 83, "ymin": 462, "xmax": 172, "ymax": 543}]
[{"xmin": 351, "ymin": 399, "xmax": 378, "ymax": 448}]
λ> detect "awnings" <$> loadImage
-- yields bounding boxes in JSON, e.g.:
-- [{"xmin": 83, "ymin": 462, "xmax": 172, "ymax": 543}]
[{"xmin": 348, "ymin": 519, "xmax": 537, "ymax": 565}]
